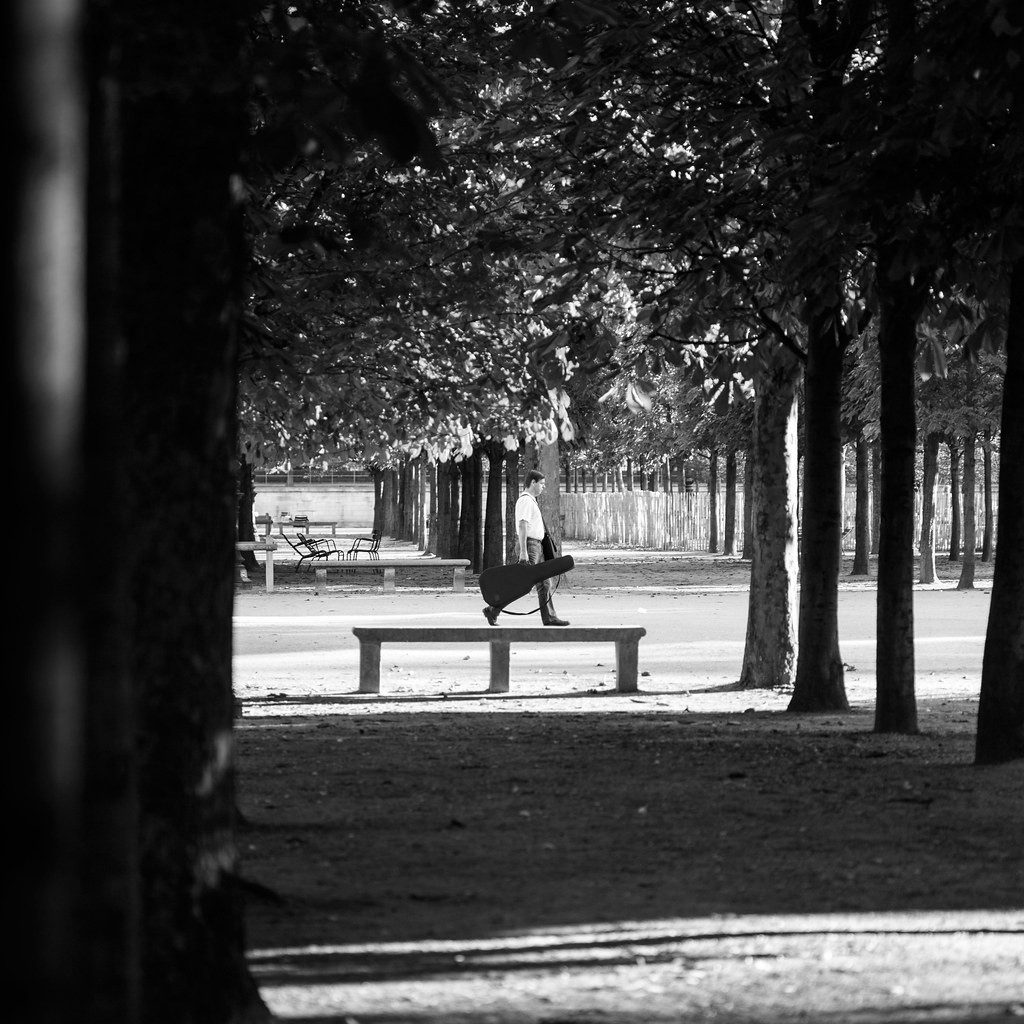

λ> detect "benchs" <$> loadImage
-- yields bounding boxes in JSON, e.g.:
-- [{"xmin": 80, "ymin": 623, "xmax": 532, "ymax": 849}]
[
  {"xmin": 256, "ymin": 513, "xmax": 273, "ymax": 535},
  {"xmin": 278, "ymin": 520, "xmax": 337, "ymax": 536},
  {"xmin": 353, "ymin": 627, "xmax": 647, "ymax": 693},
  {"xmin": 309, "ymin": 559, "xmax": 471, "ymax": 595},
  {"xmin": 236, "ymin": 542, "xmax": 278, "ymax": 592}
]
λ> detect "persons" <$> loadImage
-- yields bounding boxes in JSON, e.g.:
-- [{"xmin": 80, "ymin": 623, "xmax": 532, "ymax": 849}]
[{"xmin": 483, "ymin": 470, "xmax": 570, "ymax": 626}]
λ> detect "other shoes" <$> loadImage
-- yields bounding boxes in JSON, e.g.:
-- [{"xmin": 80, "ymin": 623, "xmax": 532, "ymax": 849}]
[
  {"xmin": 544, "ymin": 618, "xmax": 570, "ymax": 626},
  {"xmin": 482, "ymin": 607, "xmax": 497, "ymax": 625}
]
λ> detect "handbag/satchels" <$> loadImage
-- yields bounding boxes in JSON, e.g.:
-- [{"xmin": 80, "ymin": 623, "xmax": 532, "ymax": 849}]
[{"xmin": 542, "ymin": 532, "xmax": 557, "ymax": 561}]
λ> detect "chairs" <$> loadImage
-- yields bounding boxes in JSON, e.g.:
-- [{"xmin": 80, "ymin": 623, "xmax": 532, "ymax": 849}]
[
  {"xmin": 293, "ymin": 516, "xmax": 309, "ymax": 533},
  {"xmin": 347, "ymin": 529, "xmax": 382, "ymax": 574},
  {"xmin": 281, "ymin": 533, "xmax": 329, "ymax": 573},
  {"xmin": 296, "ymin": 533, "xmax": 345, "ymax": 573}
]
